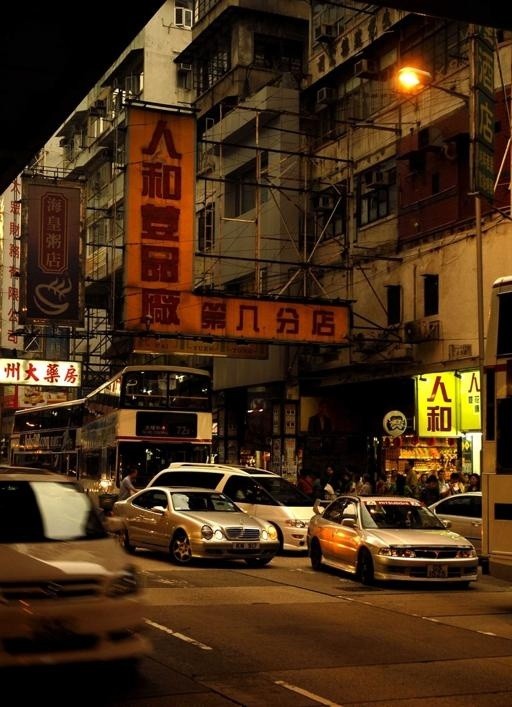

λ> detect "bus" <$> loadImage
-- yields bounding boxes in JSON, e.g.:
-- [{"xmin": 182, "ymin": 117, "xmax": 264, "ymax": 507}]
[{"xmin": 10, "ymin": 365, "xmax": 213, "ymax": 528}]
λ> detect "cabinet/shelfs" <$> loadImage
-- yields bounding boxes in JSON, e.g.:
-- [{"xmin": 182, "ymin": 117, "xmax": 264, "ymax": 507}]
[{"xmin": 380, "ymin": 439, "xmax": 461, "ymax": 480}]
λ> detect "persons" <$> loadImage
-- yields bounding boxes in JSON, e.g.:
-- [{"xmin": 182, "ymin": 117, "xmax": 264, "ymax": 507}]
[
  {"xmin": 146, "ymin": 383, "xmax": 162, "ymax": 406},
  {"xmin": 295, "ymin": 459, "xmax": 481, "ymax": 506},
  {"xmin": 119, "ymin": 467, "xmax": 140, "ymax": 500},
  {"xmin": 126, "ymin": 375, "xmax": 138, "ymax": 394}
]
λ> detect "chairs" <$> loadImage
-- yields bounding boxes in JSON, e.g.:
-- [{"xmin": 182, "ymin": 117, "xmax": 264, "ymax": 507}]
[
  {"xmin": 188, "ymin": 497, "xmax": 207, "ymax": 510},
  {"xmin": 381, "ymin": 508, "xmax": 404, "ymax": 527}
]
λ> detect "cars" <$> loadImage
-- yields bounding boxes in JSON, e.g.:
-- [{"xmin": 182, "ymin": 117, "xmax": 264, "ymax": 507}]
[{"xmin": 0, "ymin": 466, "xmax": 154, "ymax": 667}]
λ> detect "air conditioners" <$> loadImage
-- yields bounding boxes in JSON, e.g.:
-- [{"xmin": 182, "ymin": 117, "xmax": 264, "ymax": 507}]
[
  {"xmin": 365, "ymin": 169, "xmax": 390, "ymax": 190},
  {"xmin": 404, "ymin": 320, "xmax": 430, "ymax": 343},
  {"xmin": 316, "ymin": 87, "xmax": 337, "ymax": 103},
  {"xmin": 355, "ymin": 330, "xmax": 380, "ymax": 352},
  {"xmin": 314, "ymin": 191, "xmax": 335, "ymax": 209},
  {"xmin": 354, "ymin": 58, "xmax": 376, "ymax": 79},
  {"xmin": 313, "ymin": 23, "xmax": 332, "ymax": 41}
]
